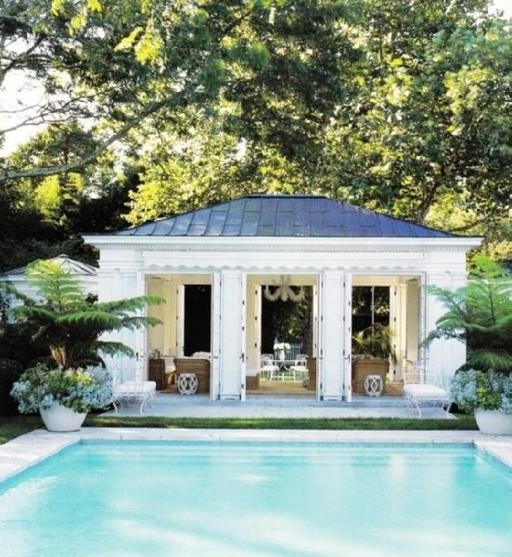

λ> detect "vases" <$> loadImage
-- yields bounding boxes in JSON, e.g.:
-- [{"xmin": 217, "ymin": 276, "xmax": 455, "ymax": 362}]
[
  {"xmin": 473, "ymin": 406, "xmax": 512, "ymax": 434},
  {"xmin": 38, "ymin": 398, "xmax": 90, "ymax": 430}
]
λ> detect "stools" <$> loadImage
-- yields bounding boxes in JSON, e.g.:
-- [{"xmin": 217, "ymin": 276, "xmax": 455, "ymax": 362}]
[
  {"xmin": 363, "ymin": 373, "xmax": 383, "ymax": 396},
  {"xmin": 177, "ymin": 372, "xmax": 199, "ymax": 395}
]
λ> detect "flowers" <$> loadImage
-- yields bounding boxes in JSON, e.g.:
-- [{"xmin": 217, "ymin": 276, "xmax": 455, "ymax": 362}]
[
  {"xmin": 448, "ymin": 368, "xmax": 512, "ymax": 415},
  {"xmin": 9, "ymin": 360, "xmax": 116, "ymax": 415}
]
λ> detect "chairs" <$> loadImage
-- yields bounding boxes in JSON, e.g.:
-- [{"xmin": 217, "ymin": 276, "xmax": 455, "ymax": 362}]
[{"xmin": 261, "ymin": 352, "xmax": 308, "ymax": 383}]
